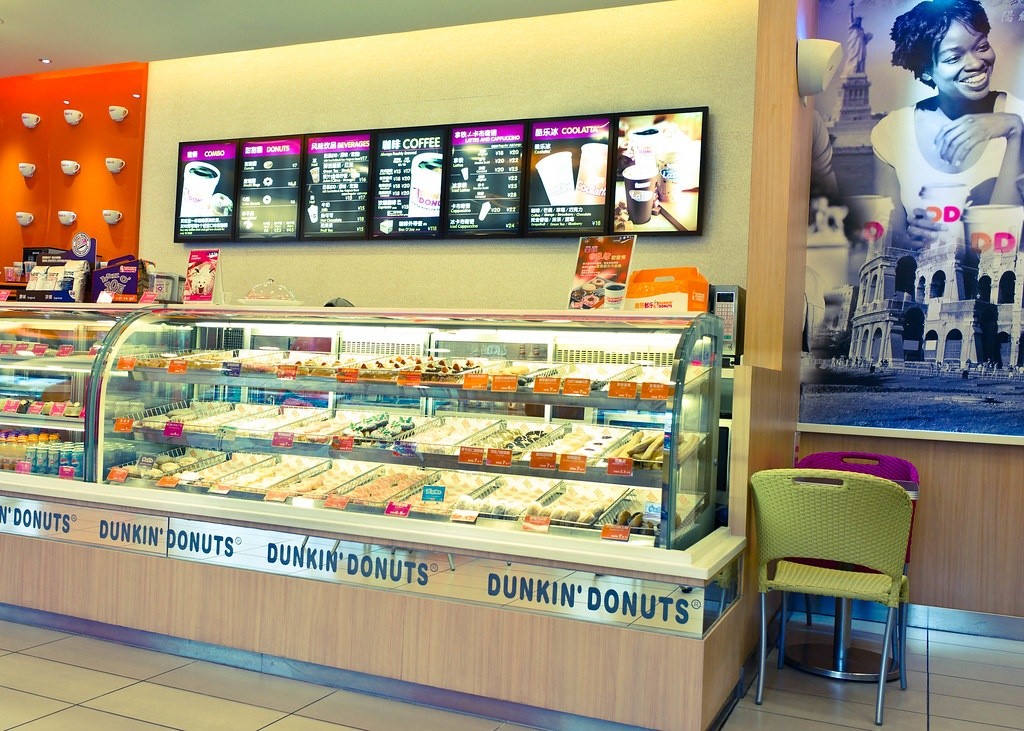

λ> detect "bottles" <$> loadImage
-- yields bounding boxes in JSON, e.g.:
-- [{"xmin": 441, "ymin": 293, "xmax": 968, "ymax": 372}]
[{"xmin": 0, "ymin": 428, "xmax": 137, "ymax": 481}]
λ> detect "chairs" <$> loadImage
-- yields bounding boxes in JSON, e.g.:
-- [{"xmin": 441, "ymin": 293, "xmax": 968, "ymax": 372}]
[
  {"xmin": 748, "ymin": 466, "xmax": 913, "ymax": 727},
  {"xmin": 785, "ymin": 449, "xmax": 921, "ymax": 658}
]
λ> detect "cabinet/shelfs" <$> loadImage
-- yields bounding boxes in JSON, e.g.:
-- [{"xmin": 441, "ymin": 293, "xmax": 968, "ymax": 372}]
[{"xmin": 0, "ymin": 296, "xmax": 726, "ymax": 550}]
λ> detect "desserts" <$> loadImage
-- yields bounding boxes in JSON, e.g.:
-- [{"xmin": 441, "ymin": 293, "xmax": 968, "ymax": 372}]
[{"xmin": 120, "ymin": 355, "xmax": 702, "ymax": 529}]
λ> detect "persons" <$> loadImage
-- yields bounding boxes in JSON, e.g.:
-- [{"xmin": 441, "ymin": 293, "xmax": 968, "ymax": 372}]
[
  {"xmin": 279, "ymin": 298, "xmax": 355, "ymax": 408},
  {"xmin": 844, "ymin": 0, "xmax": 871, "ymax": 75},
  {"xmin": 868, "ymin": 0, "xmax": 1024, "ymax": 249}
]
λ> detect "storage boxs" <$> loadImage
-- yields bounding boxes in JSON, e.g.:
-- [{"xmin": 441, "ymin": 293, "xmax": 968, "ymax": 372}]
[
  {"xmin": 625, "ymin": 266, "xmax": 710, "ymax": 311},
  {"xmin": 148, "ymin": 271, "xmax": 186, "ymax": 301}
]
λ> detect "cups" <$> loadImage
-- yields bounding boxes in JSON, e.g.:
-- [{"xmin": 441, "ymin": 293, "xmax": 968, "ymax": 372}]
[
  {"xmin": 307, "ymin": 205, "xmax": 319, "ymax": 223},
  {"xmin": 3, "ymin": 262, "xmax": 37, "ymax": 282},
  {"xmin": 15, "ymin": 158, "xmax": 125, "ymax": 225},
  {"xmin": 310, "ymin": 167, "xmax": 320, "ymax": 184},
  {"xmin": 843, "ymin": 185, "xmax": 1024, "ymax": 259},
  {"xmin": 479, "ymin": 203, "xmax": 491, "ymax": 221},
  {"xmin": 535, "ymin": 143, "xmax": 608, "ymax": 205},
  {"xmin": 180, "ymin": 162, "xmax": 221, "ymax": 217},
  {"xmin": 604, "ymin": 283, "xmax": 626, "ymax": 308},
  {"xmin": 622, "ymin": 129, "xmax": 680, "ymax": 225},
  {"xmin": 461, "ymin": 167, "xmax": 468, "ymax": 180},
  {"xmin": 21, "ymin": 105, "xmax": 129, "ymax": 129},
  {"xmin": 408, "ymin": 152, "xmax": 442, "ymax": 218}
]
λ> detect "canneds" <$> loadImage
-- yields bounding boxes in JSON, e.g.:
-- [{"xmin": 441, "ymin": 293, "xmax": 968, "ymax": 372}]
[{"xmin": 24, "ymin": 441, "xmax": 136, "ymax": 477}]
